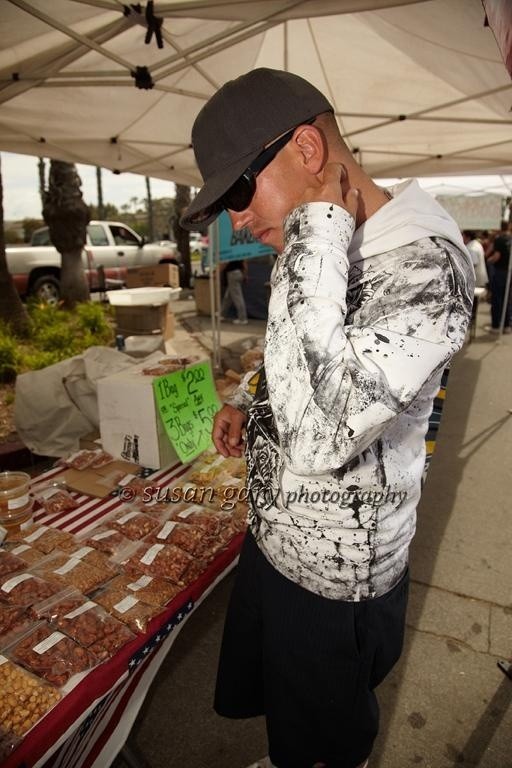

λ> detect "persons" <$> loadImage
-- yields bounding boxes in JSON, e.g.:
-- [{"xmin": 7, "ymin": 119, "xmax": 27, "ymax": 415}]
[
  {"xmin": 219, "ymin": 259, "xmax": 250, "ymax": 325},
  {"xmin": 178, "ymin": 66, "xmax": 477, "ymax": 768},
  {"xmin": 462, "ymin": 221, "xmax": 511, "ymax": 334}
]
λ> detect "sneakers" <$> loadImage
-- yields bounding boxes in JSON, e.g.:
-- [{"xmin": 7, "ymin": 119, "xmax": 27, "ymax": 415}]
[
  {"xmin": 245, "ymin": 756, "xmax": 370, "ymax": 768},
  {"xmin": 215, "ymin": 312, "xmax": 248, "ymax": 325},
  {"xmin": 484, "ymin": 325, "xmax": 511, "ymax": 335}
]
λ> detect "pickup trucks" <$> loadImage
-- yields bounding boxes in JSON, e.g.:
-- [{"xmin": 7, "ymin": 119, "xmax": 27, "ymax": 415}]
[{"xmin": 4, "ymin": 220, "xmax": 184, "ymax": 305}]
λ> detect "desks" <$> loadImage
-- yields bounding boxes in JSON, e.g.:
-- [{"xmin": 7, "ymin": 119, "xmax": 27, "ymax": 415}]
[{"xmin": 0, "ymin": 458, "xmax": 256, "ymax": 768}]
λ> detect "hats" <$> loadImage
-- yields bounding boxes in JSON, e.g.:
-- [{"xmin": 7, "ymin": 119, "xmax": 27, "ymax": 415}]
[{"xmin": 180, "ymin": 66, "xmax": 334, "ymax": 232}]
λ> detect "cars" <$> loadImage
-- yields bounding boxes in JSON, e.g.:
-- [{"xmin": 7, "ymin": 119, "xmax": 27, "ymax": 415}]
[{"xmin": 189, "ymin": 232, "xmax": 208, "ymax": 256}]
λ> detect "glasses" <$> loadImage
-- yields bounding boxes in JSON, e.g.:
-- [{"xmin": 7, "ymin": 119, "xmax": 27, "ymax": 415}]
[{"xmin": 224, "ymin": 117, "xmax": 319, "ymax": 214}]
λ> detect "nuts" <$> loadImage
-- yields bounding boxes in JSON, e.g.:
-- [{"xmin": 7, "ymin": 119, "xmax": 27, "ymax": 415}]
[
  {"xmin": 142, "ymin": 354, "xmax": 199, "ymax": 375},
  {"xmin": 0, "ymin": 446, "xmax": 248, "ymax": 765}
]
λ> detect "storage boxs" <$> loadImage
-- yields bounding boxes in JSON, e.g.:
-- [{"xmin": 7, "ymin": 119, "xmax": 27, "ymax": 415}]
[{"xmin": 106, "ymin": 263, "xmax": 181, "ymax": 350}]
